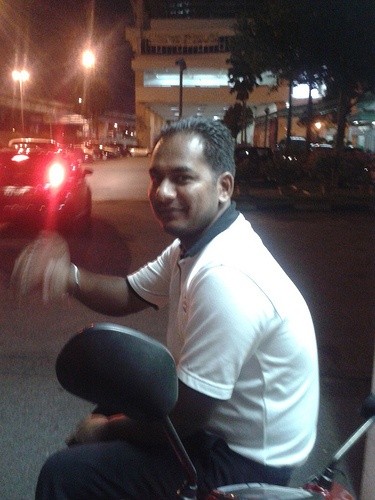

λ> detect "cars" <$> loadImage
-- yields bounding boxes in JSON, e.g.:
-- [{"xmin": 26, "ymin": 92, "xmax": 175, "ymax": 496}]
[
  {"xmin": 62, "ymin": 136, "xmax": 102, "ymax": 161},
  {"xmin": 124, "ymin": 145, "xmax": 153, "ymax": 158},
  {"xmin": 303, "ymin": 143, "xmax": 375, "ymax": 181},
  {"xmin": 234, "ymin": 146, "xmax": 276, "ymax": 182},
  {"xmin": 0, "ymin": 138, "xmax": 94, "ymax": 243}
]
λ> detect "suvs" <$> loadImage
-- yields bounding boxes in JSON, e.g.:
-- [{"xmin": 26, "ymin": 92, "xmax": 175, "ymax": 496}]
[{"xmin": 271, "ymin": 136, "xmax": 309, "ymax": 169}]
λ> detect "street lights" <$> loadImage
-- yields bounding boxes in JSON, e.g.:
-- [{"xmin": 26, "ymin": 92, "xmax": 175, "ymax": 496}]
[{"xmin": 12, "ymin": 69, "xmax": 30, "ymax": 138}]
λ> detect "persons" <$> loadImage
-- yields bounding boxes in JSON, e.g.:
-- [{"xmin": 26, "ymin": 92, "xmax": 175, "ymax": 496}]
[{"xmin": 10, "ymin": 117, "xmax": 320, "ymax": 500}]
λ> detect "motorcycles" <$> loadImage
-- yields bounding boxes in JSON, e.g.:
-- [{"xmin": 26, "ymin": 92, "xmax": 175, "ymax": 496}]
[{"xmin": 55, "ymin": 322, "xmax": 375, "ymax": 500}]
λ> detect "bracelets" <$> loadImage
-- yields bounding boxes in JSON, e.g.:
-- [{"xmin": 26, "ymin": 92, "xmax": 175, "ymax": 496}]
[{"xmin": 67, "ymin": 262, "xmax": 80, "ymax": 295}]
